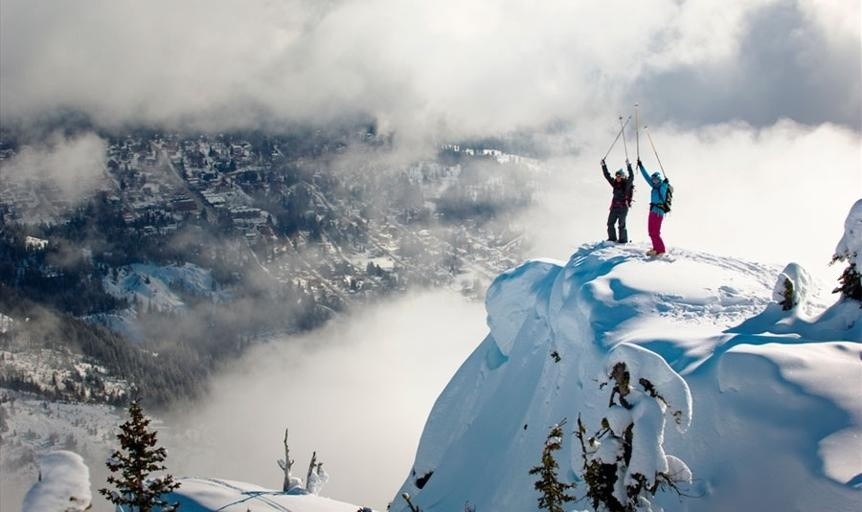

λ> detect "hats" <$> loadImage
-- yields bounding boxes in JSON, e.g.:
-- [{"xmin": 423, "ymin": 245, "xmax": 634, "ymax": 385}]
[
  {"xmin": 652, "ymin": 171, "xmax": 661, "ymax": 180},
  {"xmin": 615, "ymin": 168, "xmax": 625, "ymax": 177}
]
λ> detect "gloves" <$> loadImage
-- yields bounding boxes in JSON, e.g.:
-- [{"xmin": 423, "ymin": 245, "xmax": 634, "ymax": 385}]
[
  {"xmin": 637, "ymin": 160, "xmax": 642, "ymax": 166},
  {"xmin": 625, "ymin": 159, "xmax": 631, "ymax": 165},
  {"xmin": 600, "ymin": 160, "xmax": 606, "ymax": 166},
  {"xmin": 664, "ymin": 178, "xmax": 668, "ymax": 182}
]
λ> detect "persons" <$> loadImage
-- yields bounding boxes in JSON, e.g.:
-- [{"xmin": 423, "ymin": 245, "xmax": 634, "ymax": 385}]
[
  {"xmin": 634, "ymin": 157, "xmax": 673, "ymax": 255},
  {"xmin": 598, "ymin": 157, "xmax": 636, "ymax": 240}
]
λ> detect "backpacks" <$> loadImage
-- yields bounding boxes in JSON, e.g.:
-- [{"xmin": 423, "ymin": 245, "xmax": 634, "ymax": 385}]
[
  {"xmin": 628, "ymin": 182, "xmax": 634, "ymax": 208},
  {"xmin": 664, "ymin": 184, "xmax": 673, "ymax": 213}
]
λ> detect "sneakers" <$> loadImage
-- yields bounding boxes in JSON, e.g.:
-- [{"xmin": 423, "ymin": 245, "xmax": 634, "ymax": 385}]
[
  {"xmin": 606, "ymin": 238, "xmax": 617, "ymax": 242},
  {"xmin": 646, "ymin": 248, "xmax": 666, "ymax": 257},
  {"xmin": 616, "ymin": 239, "xmax": 628, "ymax": 244}
]
